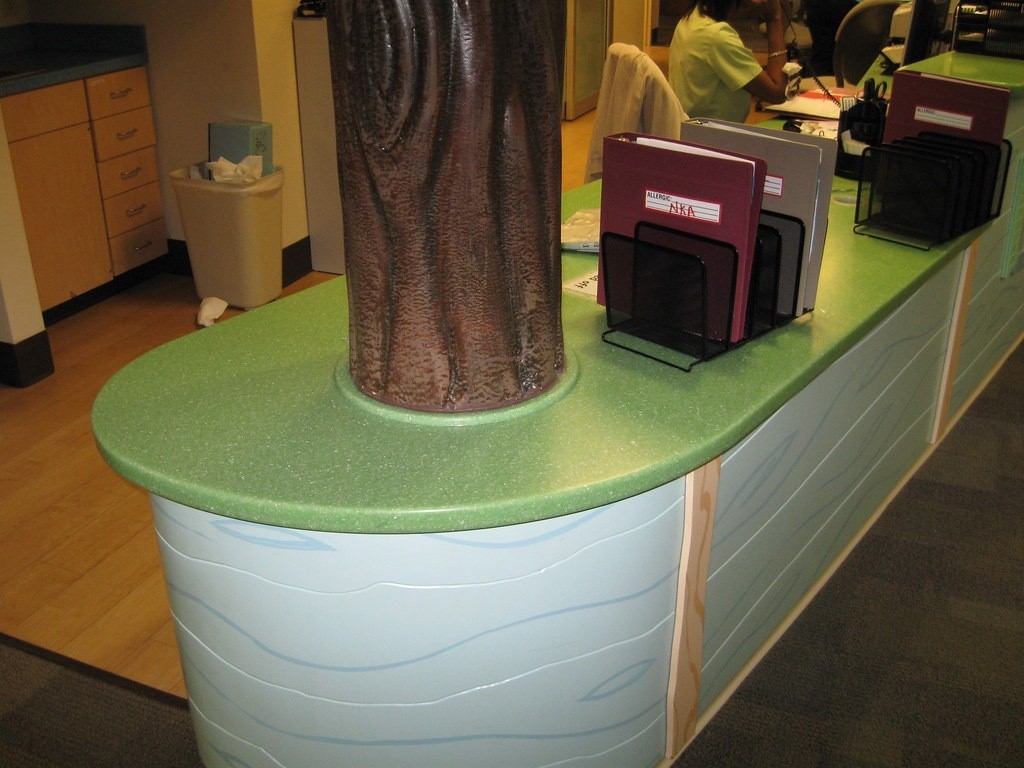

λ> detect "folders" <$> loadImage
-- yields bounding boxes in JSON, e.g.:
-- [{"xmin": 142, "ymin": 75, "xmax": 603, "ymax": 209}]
[
  {"xmin": 595, "ymin": 130, "xmax": 770, "ymax": 345},
  {"xmin": 680, "ymin": 115, "xmax": 839, "ymax": 318},
  {"xmin": 882, "ymin": 68, "xmax": 1012, "ymax": 149}
]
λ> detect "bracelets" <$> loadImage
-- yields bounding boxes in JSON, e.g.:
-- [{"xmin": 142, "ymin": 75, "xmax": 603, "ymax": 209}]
[{"xmin": 768, "ymin": 49, "xmax": 788, "ymax": 60}]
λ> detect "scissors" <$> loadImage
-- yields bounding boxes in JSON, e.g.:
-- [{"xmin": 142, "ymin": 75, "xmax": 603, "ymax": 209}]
[{"xmin": 864, "ymin": 77, "xmax": 888, "ymax": 101}]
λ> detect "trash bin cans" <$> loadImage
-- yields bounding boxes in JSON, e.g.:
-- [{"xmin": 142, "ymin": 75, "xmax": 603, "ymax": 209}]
[{"xmin": 167, "ymin": 158, "xmax": 286, "ymax": 310}]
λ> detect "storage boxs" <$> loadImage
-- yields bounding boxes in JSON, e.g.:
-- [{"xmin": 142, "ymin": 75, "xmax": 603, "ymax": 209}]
[{"xmin": 209, "ymin": 118, "xmax": 273, "ymax": 184}]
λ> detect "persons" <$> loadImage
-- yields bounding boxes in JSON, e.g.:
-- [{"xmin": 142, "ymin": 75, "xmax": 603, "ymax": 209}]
[{"xmin": 667, "ymin": 0, "xmax": 788, "ymax": 123}]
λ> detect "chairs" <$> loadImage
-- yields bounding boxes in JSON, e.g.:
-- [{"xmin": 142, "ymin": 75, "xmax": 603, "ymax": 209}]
[
  {"xmin": 792, "ymin": 0, "xmax": 900, "ymax": 93},
  {"xmin": 608, "ymin": 43, "xmax": 692, "ymax": 138}
]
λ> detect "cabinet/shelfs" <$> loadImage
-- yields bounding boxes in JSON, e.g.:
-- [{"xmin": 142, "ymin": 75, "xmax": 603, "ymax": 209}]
[
  {"xmin": 293, "ymin": 15, "xmax": 347, "ymax": 276},
  {"xmin": 0, "ymin": 64, "xmax": 167, "ymax": 323}
]
